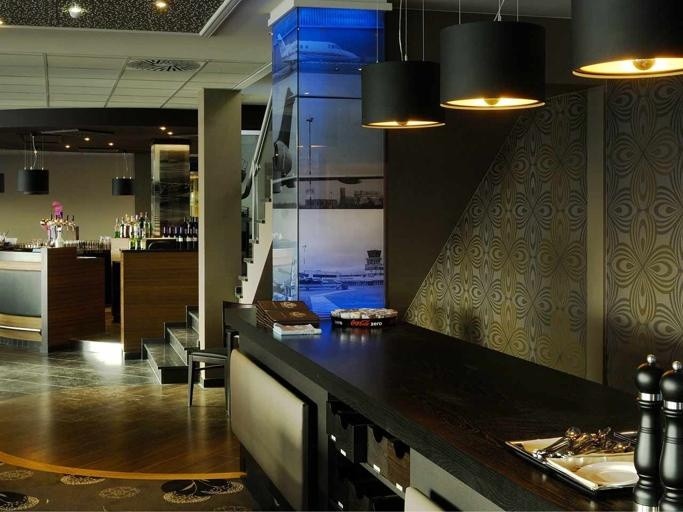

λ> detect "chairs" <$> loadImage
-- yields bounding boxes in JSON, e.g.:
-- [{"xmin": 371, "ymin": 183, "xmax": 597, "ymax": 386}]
[{"xmin": 187, "ymin": 330, "xmax": 238, "ymax": 411}]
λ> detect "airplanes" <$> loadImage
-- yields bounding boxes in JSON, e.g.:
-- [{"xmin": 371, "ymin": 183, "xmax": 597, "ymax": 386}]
[
  {"xmin": 276, "ymin": 33, "xmax": 360, "ymax": 72},
  {"xmin": 272, "ymin": 87, "xmax": 384, "ymax": 197}
]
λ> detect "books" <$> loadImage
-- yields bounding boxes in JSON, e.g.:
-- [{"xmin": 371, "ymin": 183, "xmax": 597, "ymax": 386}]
[{"xmin": 256, "ymin": 297, "xmax": 319, "ymax": 331}]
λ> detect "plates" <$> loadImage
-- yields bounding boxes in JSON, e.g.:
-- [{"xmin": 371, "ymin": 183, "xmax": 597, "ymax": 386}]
[{"xmin": 574, "ymin": 463, "xmax": 638, "ymax": 487}]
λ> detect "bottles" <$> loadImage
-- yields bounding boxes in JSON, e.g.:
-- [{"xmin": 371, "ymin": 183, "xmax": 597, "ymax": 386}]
[
  {"xmin": 129, "ymin": 239, "xmax": 147, "ymax": 251},
  {"xmin": 56, "ymin": 227, "xmax": 65, "ymax": 248},
  {"xmin": 165, "ymin": 236, "xmax": 198, "ymax": 250},
  {"xmin": 114, "ymin": 212, "xmax": 151, "ymax": 238},
  {"xmin": 163, "ymin": 217, "xmax": 199, "ymax": 236}
]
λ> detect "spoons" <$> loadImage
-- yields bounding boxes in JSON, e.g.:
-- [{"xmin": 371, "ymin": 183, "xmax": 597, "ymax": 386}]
[{"xmin": 530, "ymin": 424, "xmax": 632, "ymax": 459}]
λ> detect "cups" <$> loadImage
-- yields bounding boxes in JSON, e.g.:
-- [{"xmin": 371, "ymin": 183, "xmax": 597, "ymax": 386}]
[{"xmin": 24, "ymin": 239, "xmax": 41, "ymax": 249}]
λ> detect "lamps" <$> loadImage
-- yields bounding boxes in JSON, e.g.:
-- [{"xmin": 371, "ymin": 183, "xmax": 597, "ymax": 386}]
[
  {"xmin": 17, "ymin": 129, "xmax": 50, "ymax": 195},
  {"xmin": 361, "ymin": 1, "xmax": 446, "ymax": 129},
  {"xmin": 112, "ymin": 145, "xmax": 134, "ymax": 196},
  {"xmin": 571, "ymin": 1, "xmax": 683, "ymax": 80},
  {"xmin": 442, "ymin": 2, "xmax": 548, "ymax": 112}
]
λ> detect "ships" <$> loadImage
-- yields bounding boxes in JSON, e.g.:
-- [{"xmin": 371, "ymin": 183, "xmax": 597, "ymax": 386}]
[{"xmin": 298, "ymin": 249, "xmax": 384, "ymax": 285}]
